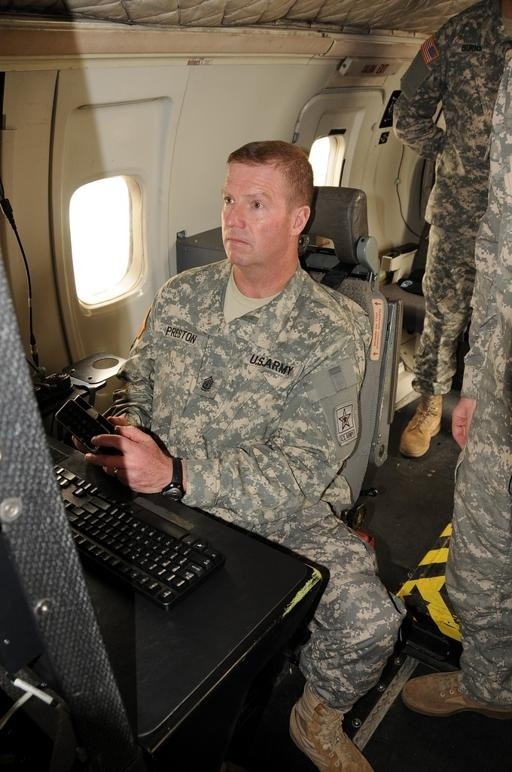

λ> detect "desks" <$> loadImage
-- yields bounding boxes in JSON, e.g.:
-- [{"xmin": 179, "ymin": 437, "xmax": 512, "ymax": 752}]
[{"xmin": 40, "ymin": 433, "xmax": 327, "ymax": 770}]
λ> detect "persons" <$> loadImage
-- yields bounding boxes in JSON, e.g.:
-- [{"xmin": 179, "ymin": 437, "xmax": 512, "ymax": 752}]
[
  {"xmin": 388, "ymin": 0, "xmax": 510, "ymax": 461},
  {"xmin": 71, "ymin": 138, "xmax": 400, "ymax": 771},
  {"xmin": 394, "ymin": 54, "xmax": 512, "ymax": 728}
]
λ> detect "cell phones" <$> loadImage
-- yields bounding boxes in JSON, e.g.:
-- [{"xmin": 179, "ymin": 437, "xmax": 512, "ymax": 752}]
[
  {"xmin": 54, "ymin": 399, "xmax": 110, "ymax": 452},
  {"xmin": 74, "ymin": 395, "xmax": 117, "ymax": 434}
]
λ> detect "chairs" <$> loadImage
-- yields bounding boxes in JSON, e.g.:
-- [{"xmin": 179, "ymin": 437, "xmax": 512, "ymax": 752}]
[{"xmin": 296, "ymin": 184, "xmax": 403, "ymax": 524}]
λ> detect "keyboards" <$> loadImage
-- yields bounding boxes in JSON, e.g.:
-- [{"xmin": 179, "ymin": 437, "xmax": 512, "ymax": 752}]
[{"xmin": 53, "ymin": 463, "xmax": 225, "ymax": 609}]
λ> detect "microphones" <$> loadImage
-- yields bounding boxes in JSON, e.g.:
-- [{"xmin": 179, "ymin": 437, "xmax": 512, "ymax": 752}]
[{"xmin": 0, "ymin": 197, "xmax": 40, "ymax": 369}]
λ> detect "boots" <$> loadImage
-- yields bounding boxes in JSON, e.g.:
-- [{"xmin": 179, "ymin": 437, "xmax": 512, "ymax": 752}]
[
  {"xmin": 286, "ymin": 682, "xmax": 375, "ymax": 772},
  {"xmin": 402, "ymin": 670, "xmax": 512, "ymax": 718},
  {"xmin": 395, "ymin": 393, "xmax": 445, "ymax": 458}
]
